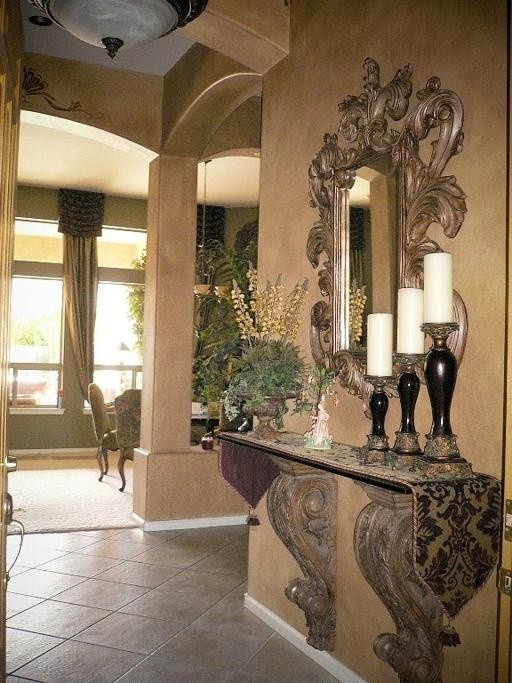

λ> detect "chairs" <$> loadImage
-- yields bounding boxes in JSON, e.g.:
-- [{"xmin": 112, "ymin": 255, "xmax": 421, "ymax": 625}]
[
  {"xmin": 89, "ymin": 383, "xmax": 119, "ymax": 480},
  {"xmin": 115, "ymin": 389, "xmax": 138, "ymax": 492}
]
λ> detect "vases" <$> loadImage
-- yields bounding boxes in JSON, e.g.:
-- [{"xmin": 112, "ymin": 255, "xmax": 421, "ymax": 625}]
[{"xmin": 238, "ymin": 394, "xmax": 298, "ymax": 438}]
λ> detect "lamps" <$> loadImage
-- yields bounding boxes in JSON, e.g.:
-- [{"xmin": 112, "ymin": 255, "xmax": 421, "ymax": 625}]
[
  {"xmin": 29, "ymin": 0, "xmax": 209, "ymax": 59},
  {"xmin": 424, "ymin": 253, "xmax": 459, "ymax": 323},
  {"xmin": 394, "ymin": 286, "xmax": 424, "ymax": 353},
  {"xmin": 366, "ymin": 312, "xmax": 396, "ymax": 378}
]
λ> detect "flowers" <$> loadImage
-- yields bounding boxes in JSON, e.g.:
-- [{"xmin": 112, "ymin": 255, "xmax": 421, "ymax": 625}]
[
  {"xmin": 349, "ymin": 280, "xmax": 367, "ymax": 351},
  {"xmin": 220, "ymin": 268, "xmax": 334, "ymax": 427}
]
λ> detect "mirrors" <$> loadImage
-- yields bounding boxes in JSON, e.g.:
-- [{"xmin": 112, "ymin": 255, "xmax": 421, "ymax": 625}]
[{"xmin": 304, "ymin": 57, "xmax": 465, "ymax": 420}]
